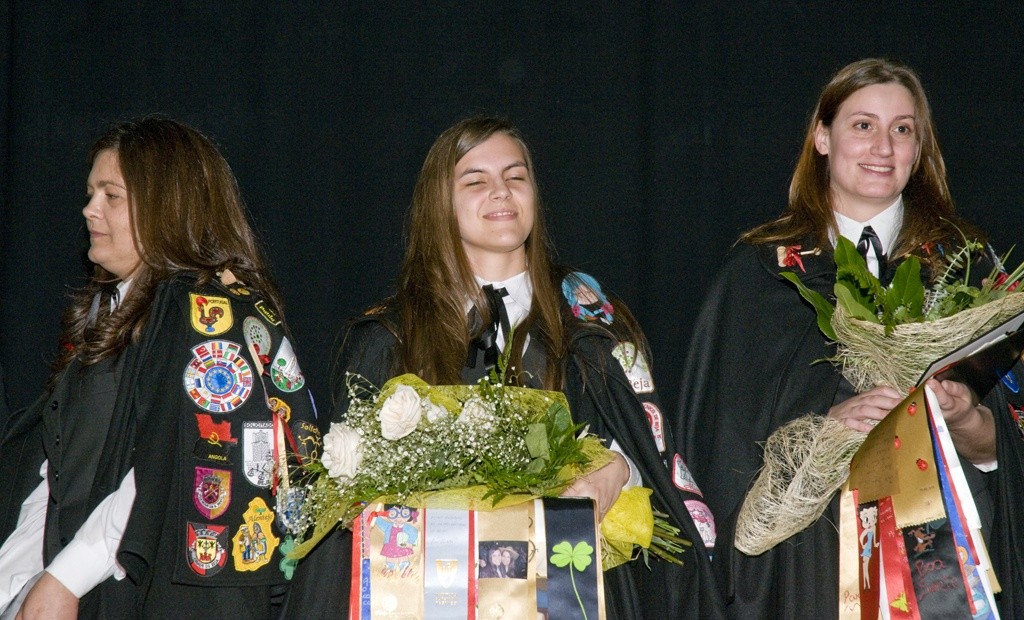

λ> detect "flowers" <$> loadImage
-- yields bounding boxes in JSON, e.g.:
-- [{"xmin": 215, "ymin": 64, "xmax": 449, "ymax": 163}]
[
  {"xmin": 269, "ymin": 323, "xmax": 692, "ymax": 567},
  {"xmin": 734, "ymin": 217, "xmax": 1024, "ymax": 557}
]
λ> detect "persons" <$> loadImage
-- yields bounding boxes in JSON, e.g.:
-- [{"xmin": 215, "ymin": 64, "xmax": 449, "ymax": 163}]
[
  {"xmin": 282, "ymin": 113, "xmax": 725, "ymax": 620},
  {"xmin": 672, "ymin": 59, "xmax": 1024, "ymax": 620},
  {"xmin": 0, "ymin": 117, "xmax": 320, "ymax": 620},
  {"xmin": 477, "ymin": 543, "xmax": 526, "ymax": 578}
]
